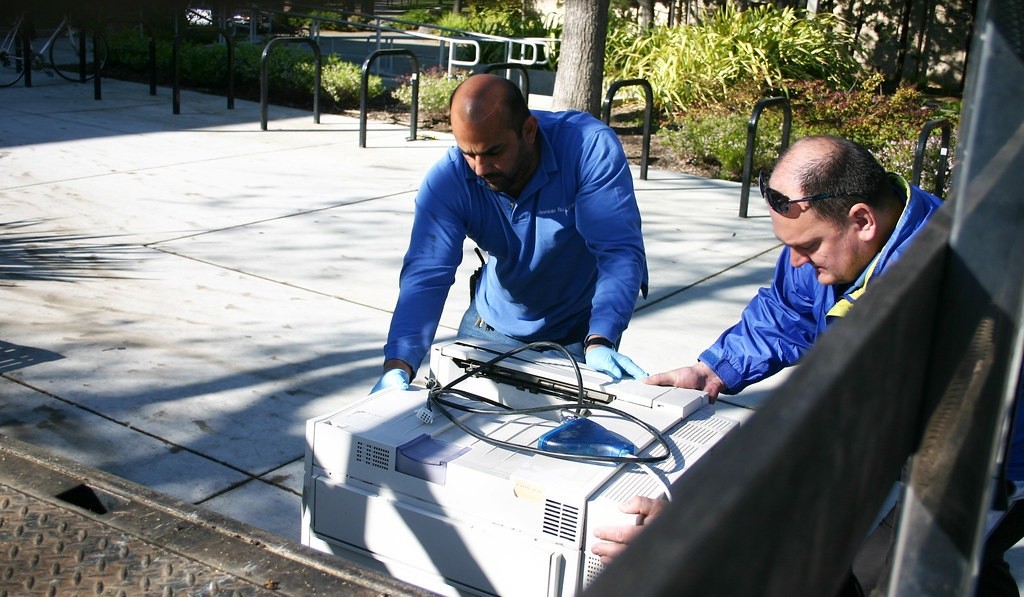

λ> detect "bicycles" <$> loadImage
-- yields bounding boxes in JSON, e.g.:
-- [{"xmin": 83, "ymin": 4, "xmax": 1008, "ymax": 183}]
[{"xmin": 0, "ymin": 8, "xmax": 110, "ymax": 87}]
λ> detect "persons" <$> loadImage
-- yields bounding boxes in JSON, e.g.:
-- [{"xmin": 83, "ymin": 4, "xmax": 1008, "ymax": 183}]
[
  {"xmin": 592, "ymin": 134, "xmax": 1024, "ymax": 597},
  {"xmin": 369, "ymin": 74, "xmax": 652, "ymax": 393}
]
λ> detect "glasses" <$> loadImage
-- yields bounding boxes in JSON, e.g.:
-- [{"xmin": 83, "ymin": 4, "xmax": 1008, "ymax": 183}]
[{"xmin": 758, "ymin": 168, "xmax": 871, "ymax": 217}]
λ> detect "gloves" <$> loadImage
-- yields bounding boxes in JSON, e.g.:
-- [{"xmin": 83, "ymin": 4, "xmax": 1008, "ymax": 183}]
[
  {"xmin": 369, "ymin": 368, "xmax": 412, "ymax": 394},
  {"xmin": 583, "ymin": 347, "xmax": 652, "ymax": 380}
]
